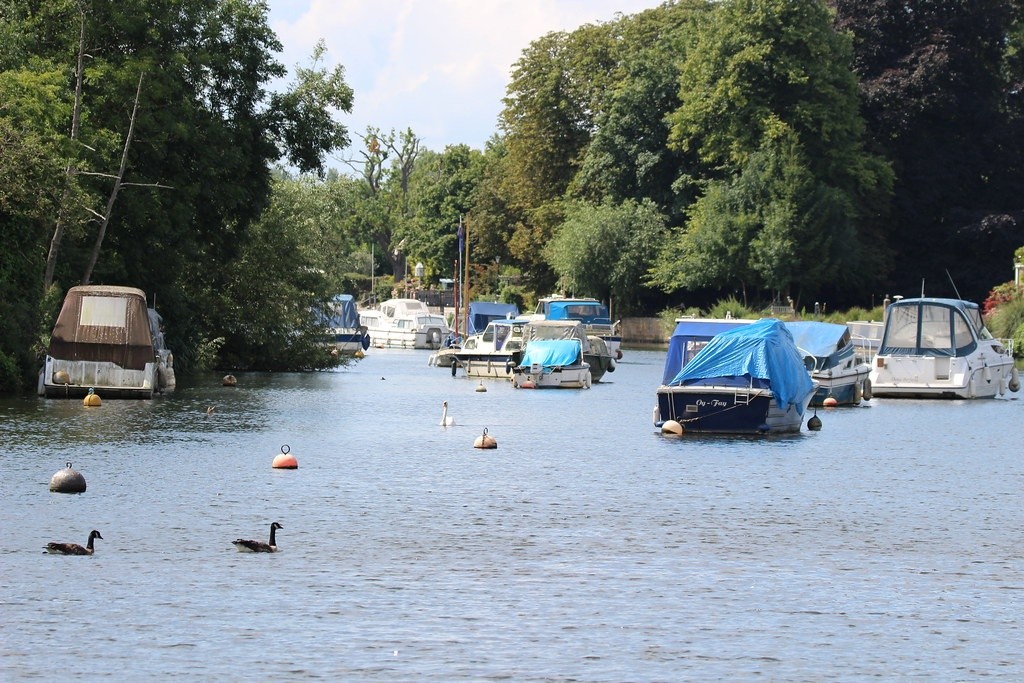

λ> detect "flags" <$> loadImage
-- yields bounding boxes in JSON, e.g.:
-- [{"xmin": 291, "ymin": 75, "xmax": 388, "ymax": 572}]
[{"xmin": 458, "ymin": 224, "xmax": 463, "ymax": 252}]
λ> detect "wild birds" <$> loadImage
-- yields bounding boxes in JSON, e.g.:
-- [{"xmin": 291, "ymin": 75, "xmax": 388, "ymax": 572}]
[
  {"xmin": 206, "ymin": 406, "xmax": 218, "ymax": 416},
  {"xmin": 41, "ymin": 528, "xmax": 104, "ymax": 557},
  {"xmin": 231, "ymin": 521, "xmax": 285, "ymax": 553}
]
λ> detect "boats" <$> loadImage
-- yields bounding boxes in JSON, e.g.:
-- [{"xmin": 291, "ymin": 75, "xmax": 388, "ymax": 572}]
[
  {"xmin": 652, "ymin": 316, "xmax": 822, "ymax": 440},
  {"xmin": 37, "ymin": 284, "xmax": 176, "ymax": 401},
  {"xmin": 284, "ymin": 210, "xmax": 624, "ymax": 390},
  {"xmin": 859, "ymin": 267, "xmax": 1015, "ymax": 402},
  {"xmin": 662, "ymin": 312, "xmax": 873, "ymax": 410}
]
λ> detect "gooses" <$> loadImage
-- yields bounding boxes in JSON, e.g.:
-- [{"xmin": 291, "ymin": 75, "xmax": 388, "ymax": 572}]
[{"xmin": 437, "ymin": 401, "xmax": 455, "ymax": 425}]
